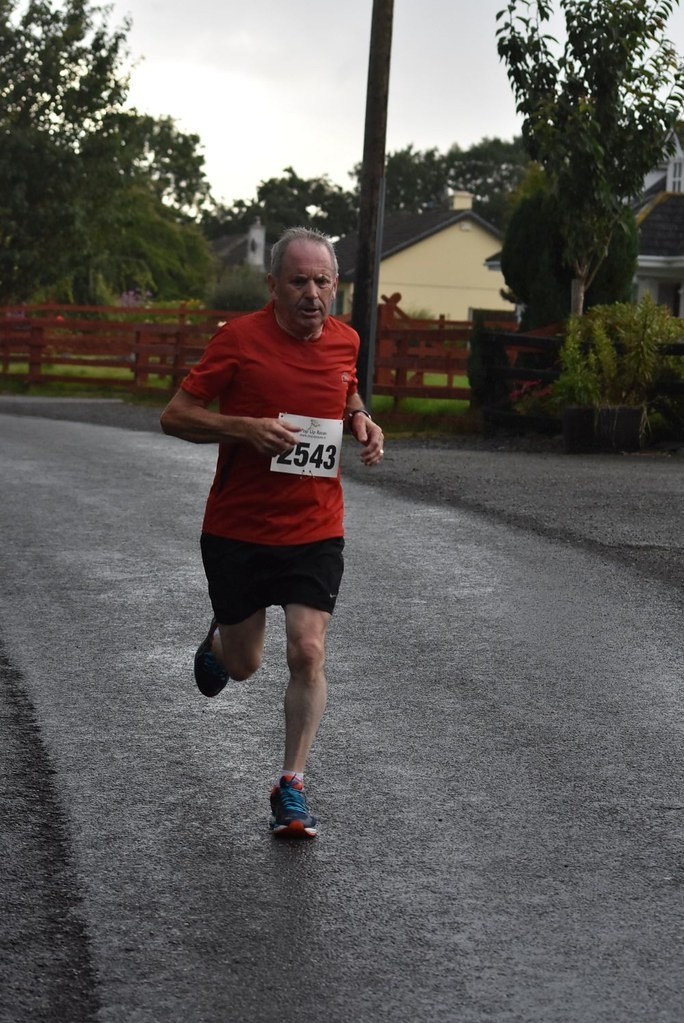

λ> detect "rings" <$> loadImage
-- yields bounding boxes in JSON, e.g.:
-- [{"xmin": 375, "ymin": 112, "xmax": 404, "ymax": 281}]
[{"xmin": 378, "ymin": 449, "xmax": 384, "ymax": 454}]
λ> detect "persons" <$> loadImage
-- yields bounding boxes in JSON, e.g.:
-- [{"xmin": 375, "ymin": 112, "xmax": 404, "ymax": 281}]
[{"xmin": 160, "ymin": 229, "xmax": 384, "ymax": 836}]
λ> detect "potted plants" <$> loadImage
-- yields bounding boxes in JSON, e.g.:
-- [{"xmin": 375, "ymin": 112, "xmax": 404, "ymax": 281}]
[{"xmin": 559, "ymin": 294, "xmax": 657, "ymax": 454}]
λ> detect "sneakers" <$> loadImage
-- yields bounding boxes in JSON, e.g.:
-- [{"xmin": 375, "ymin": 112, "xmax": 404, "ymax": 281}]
[
  {"xmin": 194, "ymin": 616, "xmax": 229, "ymax": 697},
  {"xmin": 268, "ymin": 773, "xmax": 317, "ymax": 838}
]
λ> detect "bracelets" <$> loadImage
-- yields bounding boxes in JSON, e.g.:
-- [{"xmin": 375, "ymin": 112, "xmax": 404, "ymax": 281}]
[{"xmin": 349, "ymin": 409, "xmax": 372, "ymax": 436}]
